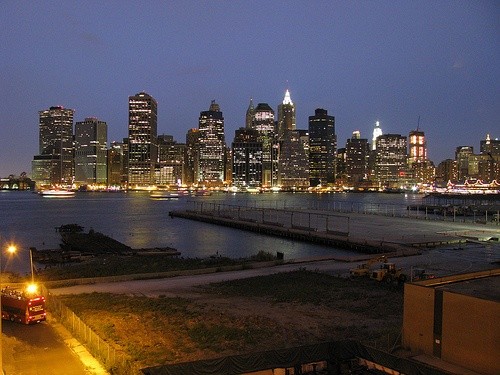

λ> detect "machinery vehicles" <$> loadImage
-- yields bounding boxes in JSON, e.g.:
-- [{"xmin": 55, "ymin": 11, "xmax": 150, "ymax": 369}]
[
  {"xmin": 350, "ymin": 256, "xmax": 388, "ymax": 277},
  {"xmin": 372, "ymin": 263, "xmax": 406, "ymax": 284}
]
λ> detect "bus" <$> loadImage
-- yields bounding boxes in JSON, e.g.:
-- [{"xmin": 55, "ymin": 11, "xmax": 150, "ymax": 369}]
[{"xmin": 1, "ymin": 289, "xmax": 46, "ymax": 325}]
